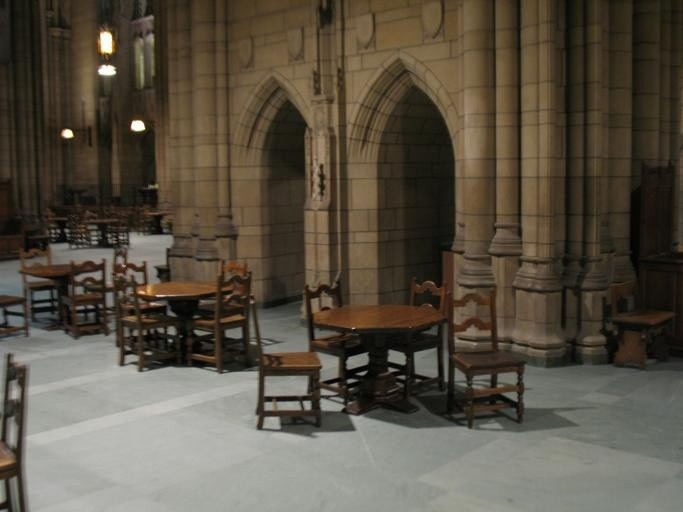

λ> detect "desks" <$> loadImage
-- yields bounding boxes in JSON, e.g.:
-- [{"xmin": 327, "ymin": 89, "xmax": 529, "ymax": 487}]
[
  {"xmin": 126, "ymin": 281, "xmax": 234, "ymax": 370},
  {"xmin": 18, "ymin": 265, "xmax": 101, "ymax": 336},
  {"xmin": 311, "ymin": 303, "xmax": 448, "ymax": 415}
]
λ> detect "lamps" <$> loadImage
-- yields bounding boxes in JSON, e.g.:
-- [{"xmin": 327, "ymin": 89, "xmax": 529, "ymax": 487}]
[
  {"xmin": 129, "ymin": 115, "xmax": 154, "ymax": 136},
  {"xmin": 94, "ymin": 0, "xmax": 117, "ymax": 77},
  {"xmin": 61, "ymin": 124, "xmax": 96, "ymax": 152}
]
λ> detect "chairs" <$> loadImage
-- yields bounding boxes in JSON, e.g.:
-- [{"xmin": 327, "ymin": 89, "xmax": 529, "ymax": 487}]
[
  {"xmin": 450, "ymin": 290, "xmax": 523, "ymax": 427},
  {"xmin": 84, "ymin": 248, "xmax": 130, "ymax": 323},
  {"xmin": 304, "ymin": 281, "xmax": 383, "ymax": 406},
  {"xmin": 385, "ymin": 275, "xmax": 449, "ymax": 396},
  {"xmin": 195, "ymin": 257, "xmax": 247, "ymax": 350},
  {"xmin": 607, "ymin": 279, "xmax": 676, "ymax": 366},
  {"xmin": 184, "ymin": 269, "xmax": 256, "ymax": 373},
  {"xmin": 18, "ymin": 248, "xmax": 61, "ymax": 324},
  {"xmin": 250, "ymin": 295, "xmax": 323, "ymax": 424},
  {"xmin": 1, "ymin": 350, "xmax": 30, "ymax": 511},
  {"xmin": 112, "ymin": 260, "xmax": 171, "ymax": 346},
  {"xmin": 111, "ymin": 274, "xmax": 184, "ymax": 375},
  {"xmin": 0, "ymin": 293, "xmax": 30, "ymax": 340},
  {"xmin": 64, "ymin": 257, "xmax": 108, "ymax": 338}
]
